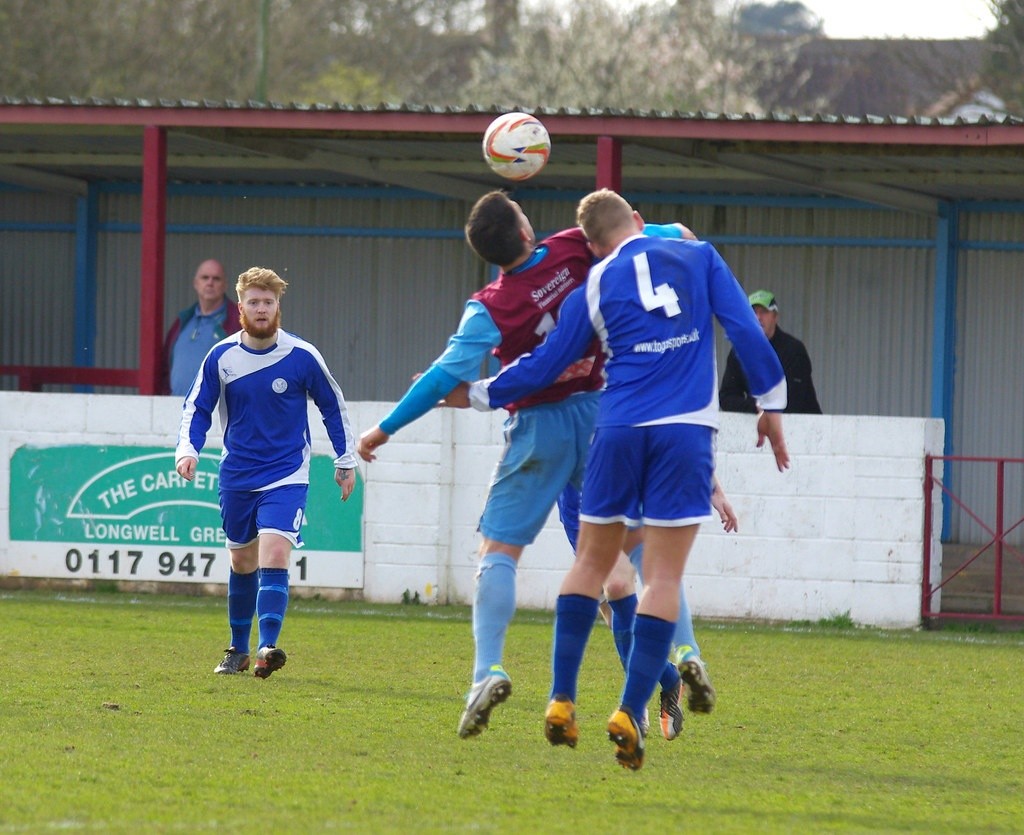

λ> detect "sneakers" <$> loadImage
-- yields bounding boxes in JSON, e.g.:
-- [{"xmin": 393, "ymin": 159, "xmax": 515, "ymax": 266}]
[
  {"xmin": 606, "ymin": 707, "xmax": 643, "ymax": 772},
  {"xmin": 659, "ymin": 665, "xmax": 684, "ymax": 742},
  {"xmin": 676, "ymin": 645, "xmax": 715, "ymax": 714},
  {"xmin": 214, "ymin": 645, "xmax": 250, "ymax": 675},
  {"xmin": 543, "ymin": 692, "xmax": 577, "ymax": 748},
  {"xmin": 641, "ymin": 709, "xmax": 650, "ymax": 736},
  {"xmin": 254, "ymin": 645, "xmax": 286, "ymax": 679},
  {"xmin": 458, "ymin": 665, "xmax": 512, "ymax": 740}
]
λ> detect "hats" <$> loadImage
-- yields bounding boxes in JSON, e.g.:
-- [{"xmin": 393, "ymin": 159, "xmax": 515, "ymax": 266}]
[{"xmin": 748, "ymin": 289, "xmax": 778, "ymax": 312}]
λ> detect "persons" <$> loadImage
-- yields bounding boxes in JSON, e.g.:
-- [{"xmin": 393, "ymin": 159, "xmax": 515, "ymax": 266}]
[
  {"xmin": 163, "ymin": 258, "xmax": 244, "ymax": 396},
  {"xmin": 719, "ymin": 290, "xmax": 823, "ymax": 414},
  {"xmin": 175, "ymin": 267, "xmax": 358, "ymax": 679},
  {"xmin": 359, "ymin": 191, "xmax": 791, "ymax": 772}
]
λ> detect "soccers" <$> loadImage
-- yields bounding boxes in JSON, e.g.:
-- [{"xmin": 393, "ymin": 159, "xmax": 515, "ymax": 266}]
[{"xmin": 483, "ymin": 112, "xmax": 550, "ymax": 179}]
